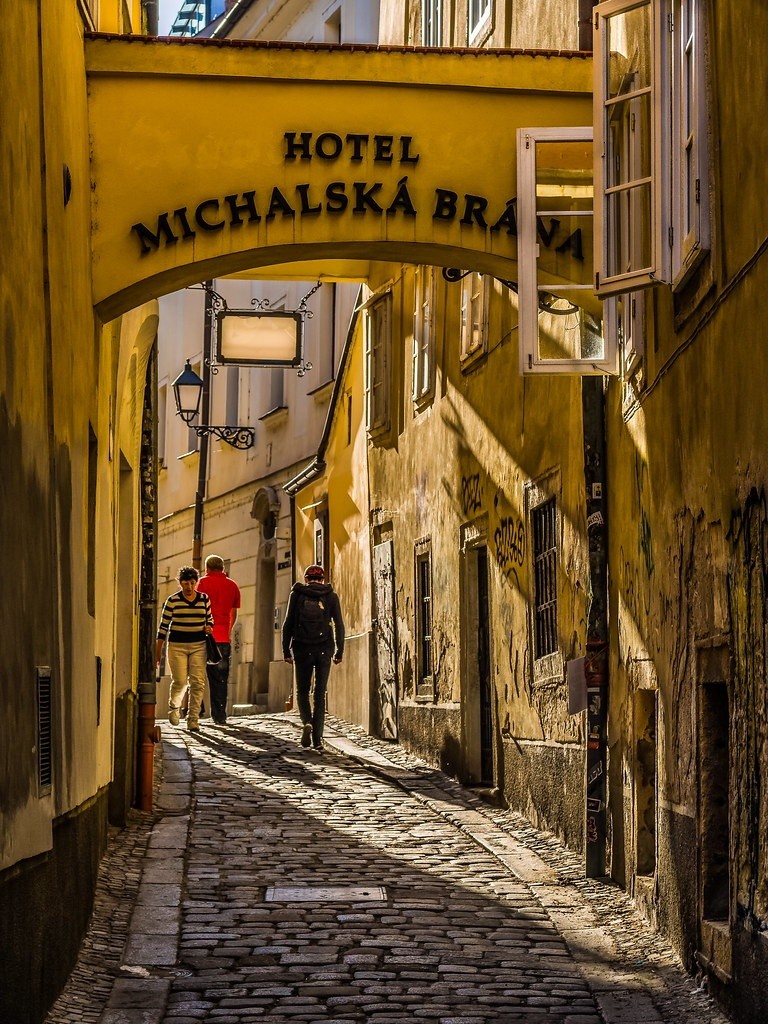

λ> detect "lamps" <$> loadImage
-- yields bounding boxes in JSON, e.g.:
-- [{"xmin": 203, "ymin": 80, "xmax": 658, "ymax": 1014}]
[{"xmin": 171, "ymin": 358, "xmax": 256, "ymax": 451}]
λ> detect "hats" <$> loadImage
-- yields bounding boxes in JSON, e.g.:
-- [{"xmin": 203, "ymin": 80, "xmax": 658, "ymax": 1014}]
[{"xmin": 304, "ymin": 565, "xmax": 325, "ymax": 578}]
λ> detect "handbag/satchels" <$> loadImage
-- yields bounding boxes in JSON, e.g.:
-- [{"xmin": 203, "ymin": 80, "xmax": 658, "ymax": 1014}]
[{"xmin": 206, "ymin": 634, "xmax": 222, "ymax": 665}]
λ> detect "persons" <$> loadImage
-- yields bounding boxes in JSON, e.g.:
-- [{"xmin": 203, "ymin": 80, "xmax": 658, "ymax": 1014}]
[
  {"xmin": 197, "ymin": 555, "xmax": 241, "ymax": 724},
  {"xmin": 282, "ymin": 565, "xmax": 345, "ymax": 751},
  {"xmin": 155, "ymin": 567, "xmax": 214, "ymax": 733}
]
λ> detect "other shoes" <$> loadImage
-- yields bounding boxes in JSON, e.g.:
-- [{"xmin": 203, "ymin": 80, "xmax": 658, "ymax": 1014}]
[
  {"xmin": 187, "ymin": 717, "xmax": 199, "ymax": 731},
  {"xmin": 168, "ymin": 707, "xmax": 179, "ymax": 726},
  {"xmin": 313, "ymin": 741, "xmax": 324, "ymax": 750},
  {"xmin": 301, "ymin": 723, "xmax": 312, "ymax": 748}
]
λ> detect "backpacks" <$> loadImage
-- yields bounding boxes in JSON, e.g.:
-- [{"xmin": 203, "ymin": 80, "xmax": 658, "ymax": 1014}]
[{"xmin": 295, "ymin": 593, "xmax": 329, "ymax": 644}]
